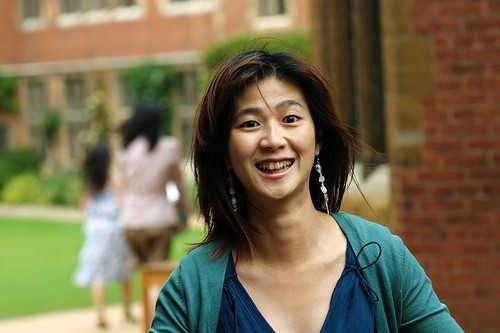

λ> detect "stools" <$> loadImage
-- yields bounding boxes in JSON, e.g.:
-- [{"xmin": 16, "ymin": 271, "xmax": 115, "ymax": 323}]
[{"xmin": 140, "ymin": 263, "xmax": 179, "ymax": 333}]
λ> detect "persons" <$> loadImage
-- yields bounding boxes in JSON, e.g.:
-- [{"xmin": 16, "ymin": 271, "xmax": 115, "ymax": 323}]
[
  {"xmin": 73, "ymin": 141, "xmax": 139, "ymax": 329},
  {"xmin": 116, "ymin": 101, "xmax": 191, "ymax": 263},
  {"xmin": 146, "ymin": 51, "xmax": 466, "ymax": 332}
]
